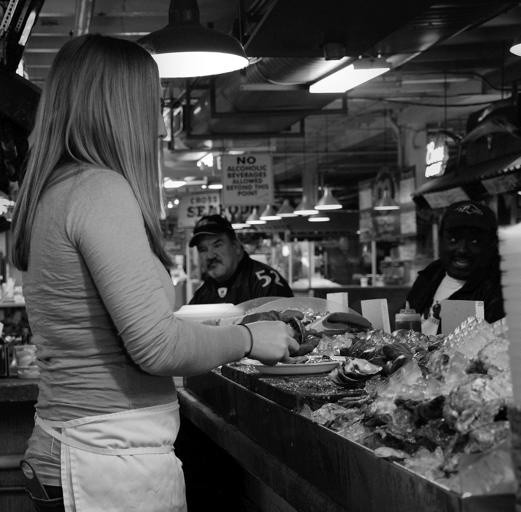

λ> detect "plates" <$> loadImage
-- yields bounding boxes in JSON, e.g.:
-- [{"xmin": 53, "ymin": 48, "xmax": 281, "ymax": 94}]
[{"xmin": 253, "ymin": 361, "xmax": 341, "ymax": 374}]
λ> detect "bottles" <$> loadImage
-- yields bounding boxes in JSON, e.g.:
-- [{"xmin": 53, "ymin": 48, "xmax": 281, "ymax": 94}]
[{"xmin": 395, "ymin": 302, "xmax": 422, "ymax": 333}]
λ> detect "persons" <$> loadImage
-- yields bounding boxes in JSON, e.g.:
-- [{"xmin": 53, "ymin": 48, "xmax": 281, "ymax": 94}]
[
  {"xmin": 403, "ymin": 199, "xmax": 507, "ymax": 327},
  {"xmin": 3, "ymin": 30, "xmax": 303, "ymax": 512},
  {"xmin": 183, "ymin": 214, "xmax": 295, "ymax": 305}
]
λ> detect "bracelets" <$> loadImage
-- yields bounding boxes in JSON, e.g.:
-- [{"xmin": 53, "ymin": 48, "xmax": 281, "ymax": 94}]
[{"xmin": 238, "ymin": 322, "xmax": 254, "ymax": 356}]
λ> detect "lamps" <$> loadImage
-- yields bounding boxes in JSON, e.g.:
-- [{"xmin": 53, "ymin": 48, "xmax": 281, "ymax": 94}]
[
  {"xmin": 309, "ymin": 58, "xmax": 392, "ymax": 94},
  {"xmin": 374, "ymin": 87, "xmax": 401, "ymax": 211},
  {"xmin": 137, "ymin": 0, "xmax": 250, "ymax": 78},
  {"xmin": 229, "ymin": 112, "xmax": 344, "ymax": 228}
]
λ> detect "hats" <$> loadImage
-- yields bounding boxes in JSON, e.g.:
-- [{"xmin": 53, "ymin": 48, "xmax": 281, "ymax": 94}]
[{"xmin": 189, "ymin": 215, "xmax": 232, "ymax": 247}]
